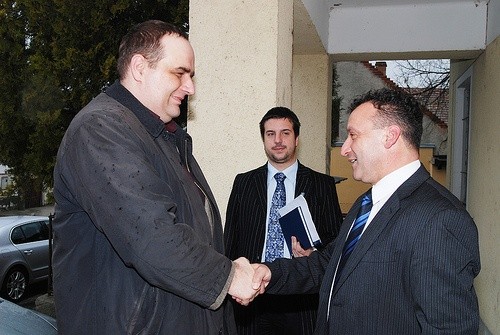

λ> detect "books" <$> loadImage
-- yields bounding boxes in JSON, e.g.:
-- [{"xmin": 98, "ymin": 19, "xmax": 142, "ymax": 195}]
[{"xmin": 278, "ymin": 192, "xmax": 322, "ymax": 255}]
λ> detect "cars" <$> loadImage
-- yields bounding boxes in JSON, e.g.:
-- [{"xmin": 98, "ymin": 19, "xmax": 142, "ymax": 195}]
[{"xmin": 0, "ymin": 215, "xmax": 54, "ymax": 303}]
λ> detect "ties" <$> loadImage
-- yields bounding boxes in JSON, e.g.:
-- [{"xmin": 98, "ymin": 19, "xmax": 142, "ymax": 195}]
[
  {"xmin": 335, "ymin": 195, "xmax": 373, "ymax": 289},
  {"xmin": 265, "ymin": 173, "xmax": 286, "ymax": 265}
]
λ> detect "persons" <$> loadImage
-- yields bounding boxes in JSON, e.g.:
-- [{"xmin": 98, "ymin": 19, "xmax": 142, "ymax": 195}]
[
  {"xmin": 224, "ymin": 107, "xmax": 344, "ymax": 335},
  {"xmin": 233, "ymin": 87, "xmax": 480, "ymax": 334},
  {"xmin": 52, "ymin": 20, "xmax": 265, "ymax": 335}
]
